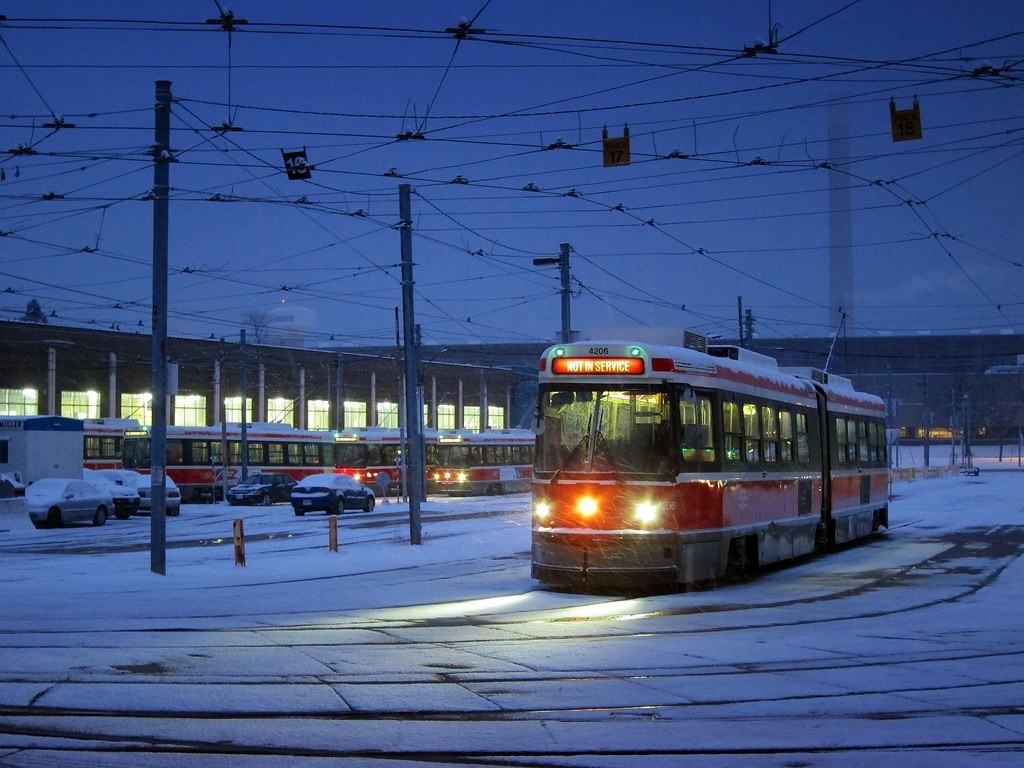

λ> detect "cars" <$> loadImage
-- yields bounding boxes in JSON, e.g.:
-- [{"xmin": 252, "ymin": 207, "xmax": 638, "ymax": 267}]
[
  {"xmin": 81, "ymin": 467, "xmax": 141, "ymax": 520},
  {"xmin": 23, "ymin": 478, "xmax": 116, "ymax": 529},
  {"xmin": 97, "ymin": 468, "xmax": 183, "ymax": 517},
  {"xmin": 225, "ymin": 472, "xmax": 301, "ymax": 506},
  {"xmin": 290, "ymin": 473, "xmax": 376, "ymax": 515}
]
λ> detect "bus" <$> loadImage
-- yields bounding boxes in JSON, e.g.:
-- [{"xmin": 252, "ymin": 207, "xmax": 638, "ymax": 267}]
[
  {"xmin": 82, "ymin": 416, "xmax": 537, "ymax": 500},
  {"xmin": 527, "ymin": 337, "xmax": 895, "ymax": 586}
]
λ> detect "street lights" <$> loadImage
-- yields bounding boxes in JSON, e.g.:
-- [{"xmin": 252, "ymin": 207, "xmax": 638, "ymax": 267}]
[{"xmin": 531, "ymin": 241, "xmax": 572, "ymax": 346}]
